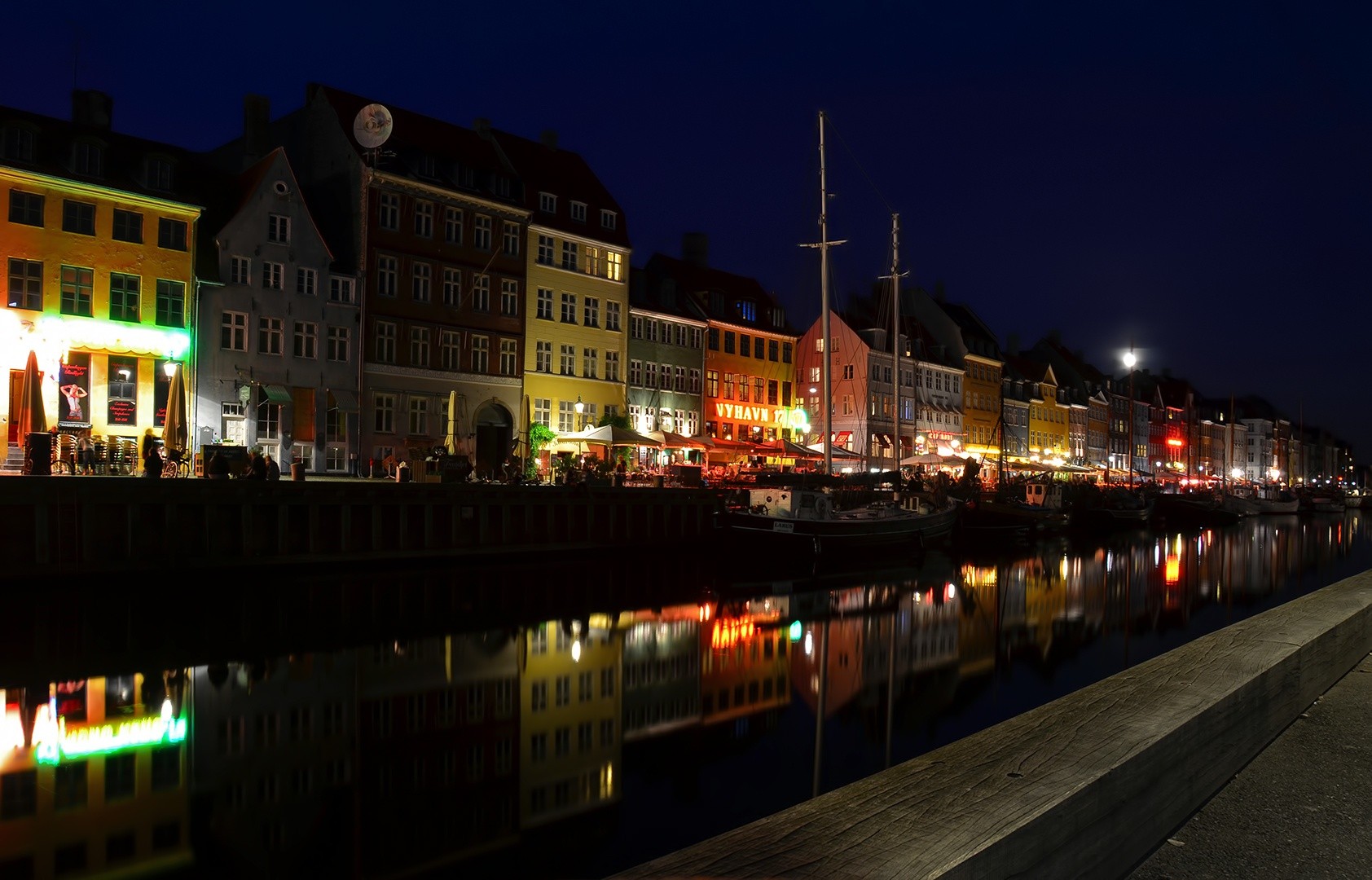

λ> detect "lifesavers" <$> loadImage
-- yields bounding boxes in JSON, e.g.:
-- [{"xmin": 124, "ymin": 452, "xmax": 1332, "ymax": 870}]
[{"xmin": 815, "ymin": 497, "xmax": 828, "ymax": 515}]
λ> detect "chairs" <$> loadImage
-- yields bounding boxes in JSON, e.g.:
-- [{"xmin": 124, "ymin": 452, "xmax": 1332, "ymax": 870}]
[
  {"xmin": 626, "ymin": 471, "xmax": 678, "ymax": 487},
  {"xmin": 51, "ymin": 432, "xmax": 139, "ymax": 477}
]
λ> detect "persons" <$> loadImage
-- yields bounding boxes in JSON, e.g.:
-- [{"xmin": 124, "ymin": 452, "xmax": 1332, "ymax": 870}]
[
  {"xmin": 903, "ymin": 468, "xmax": 1276, "ymax": 497},
  {"xmin": 702, "ymin": 460, "xmax": 768, "ymax": 482},
  {"xmin": 637, "ymin": 461, "xmax": 660, "ymax": 480},
  {"xmin": 79, "ymin": 433, "xmax": 95, "ymax": 475},
  {"xmin": 143, "ymin": 428, "xmax": 162, "ymax": 478},
  {"xmin": 247, "ymin": 451, "xmax": 280, "ymax": 480},
  {"xmin": 610, "ymin": 454, "xmax": 631, "ymax": 481}
]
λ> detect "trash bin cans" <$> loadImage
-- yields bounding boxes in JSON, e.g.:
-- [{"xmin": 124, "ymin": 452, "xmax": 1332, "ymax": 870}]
[
  {"xmin": 23, "ymin": 432, "xmax": 51, "ymax": 475},
  {"xmin": 396, "ymin": 467, "xmax": 410, "ymax": 482}
]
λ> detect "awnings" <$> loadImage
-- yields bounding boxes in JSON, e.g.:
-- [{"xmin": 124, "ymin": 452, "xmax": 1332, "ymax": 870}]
[
  {"xmin": 328, "ymin": 387, "xmax": 349, "ymax": 411},
  {"xmin": 259, "ymin": 385, "xmax": 293, "ymax": 408}
]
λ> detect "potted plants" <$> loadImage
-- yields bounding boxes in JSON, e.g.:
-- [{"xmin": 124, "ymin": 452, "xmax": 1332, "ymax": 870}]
[{"xmin": 552, "ymin": 459, "xmax": 564, "ymax": 484}]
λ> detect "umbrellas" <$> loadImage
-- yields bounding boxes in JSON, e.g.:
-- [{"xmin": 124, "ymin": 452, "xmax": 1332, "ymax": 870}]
[
  {"xmin": 512, "ymin": 394, "xmax": 530, "ymax": 475},
  {"xmin": 899, "ymin": 454, "xmax": 1245, "ymax": 482},
  {"xmin": 444, "ymin": 391, "xmax": 462, "ymax": 455},
  {"xmin": 558, "ymin": 424, "xmax": 869, "ymax": 474},
  {"xmin": 18, "ymin": 350, "xmax": 47, "ymax": 447},
  {"xmin": 162, "ymin": 364, "xmax": 188, "ymax": 462}
]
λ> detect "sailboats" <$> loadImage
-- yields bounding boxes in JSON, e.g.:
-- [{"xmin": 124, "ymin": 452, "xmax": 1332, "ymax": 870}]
[{"xmin": 708, "ymin": 103, "xmax": 1371, "ymax": 539}]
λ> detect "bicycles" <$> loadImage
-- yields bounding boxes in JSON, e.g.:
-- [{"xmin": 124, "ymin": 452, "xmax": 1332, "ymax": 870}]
[{"xmin": 159, "ymin": 443, "xmax": 191, "ymax": 478}]
